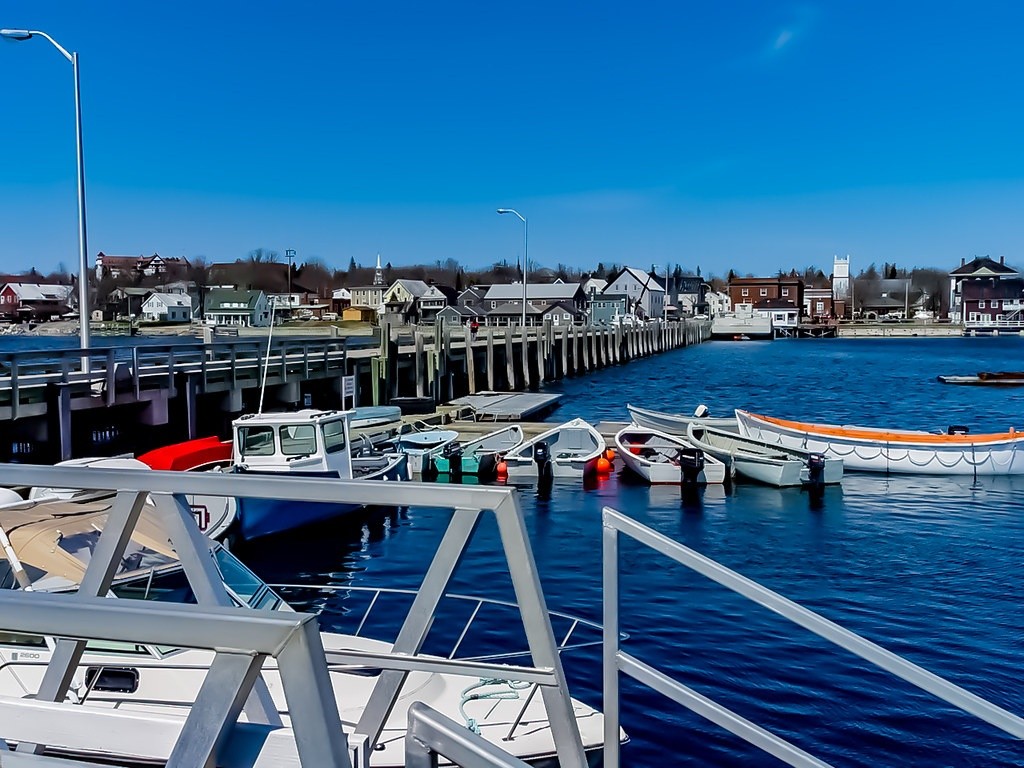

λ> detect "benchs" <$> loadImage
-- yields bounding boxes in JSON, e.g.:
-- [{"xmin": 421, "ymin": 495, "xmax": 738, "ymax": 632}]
[
  {"xmin": 462, "ymin": 448, "xmax": 498, "ymax": 451},
  {"xmin": 623, "ymin": 444, "xmax": 683, "ymax": 449},
  {"xmin": 754, "ymin": 452, "xmax": 790, "ymax": 457},
  {"xmin": 555, "ymin": 447, "xmax": 591, "ymax": 454},
  {"xmin": 382, "ymin": 447, "xmax": 426, "ymax": 452}
]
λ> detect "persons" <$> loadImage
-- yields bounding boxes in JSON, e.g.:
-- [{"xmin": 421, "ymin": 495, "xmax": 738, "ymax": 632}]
[{"xmin": 466, "ymin": 317, "xmax": 480, "ymax": 341}]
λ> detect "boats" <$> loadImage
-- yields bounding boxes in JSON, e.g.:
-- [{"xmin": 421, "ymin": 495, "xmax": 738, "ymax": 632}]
[
  {"xmin": 685, "ymin": 422, "xmax": 844, "ymax": 488},
  {"xmin": 627, "ymin": 402, "xmax": 740, "ymax": 441},
  {"xmin": 401, "ymin": 430, "xmax": 460, "ymax": 473},
  {"xmin": 613, "ymin": 421, "xmax": 726, "ymax": 485},
  {"xmin": 937, "ymin": 370, "xmax": 1024, "ymax": 386},
  {"xmin": 0, "ymin": 487, "xmax": 632, "ymax": 768},
  {"xmin": 733, "ymin": 408, "xmax": 1024, "ymax": 475},
  {"xmin": 28, "ymin": 300, "xmax": 407, "ymax": 544},
  {"xmin": 502, "ymin": 416, "xmax": 607, "ymax": 478},
  {"xmin": 431, "ymin": 425, "xmax": 524, "ymax": 474}
]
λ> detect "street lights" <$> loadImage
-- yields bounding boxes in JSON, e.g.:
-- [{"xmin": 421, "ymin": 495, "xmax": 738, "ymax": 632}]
[
  {"xmin": 497, "ymin": 208, "xmax": 527, "ymax": 326},
  {"xmin": 654, "ymin": 264, "xmax": 668, "ymax": 329},
  {"xmin": 0, "ymin": 28, "xmax": 90, "ymax": 371}
]
